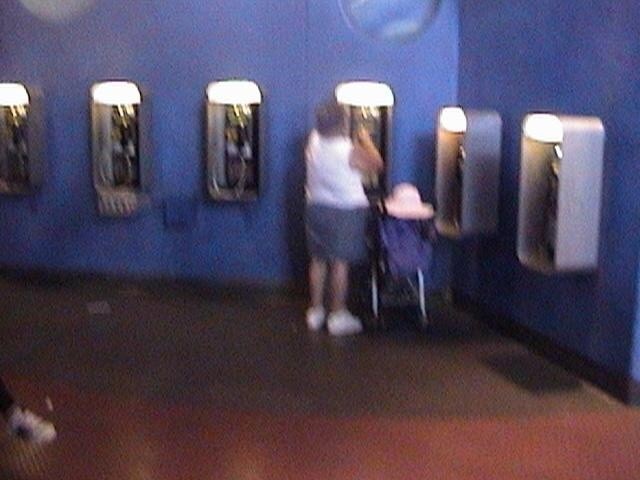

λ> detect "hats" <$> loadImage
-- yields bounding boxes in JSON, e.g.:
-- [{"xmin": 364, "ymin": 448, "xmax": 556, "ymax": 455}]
[{"xmin": 378, "ymin": 183, "xmax": 434, "ymax": 221}]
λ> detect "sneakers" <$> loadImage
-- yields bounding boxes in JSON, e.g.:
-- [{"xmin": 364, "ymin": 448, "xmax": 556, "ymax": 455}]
[
  {"xmin": 306, "ymin": 306, "xmax": 323, "ymax": 331},
  {"xmin": 13, "ymin": 414, "xmax": 57, "ymax": 448},
  {"xmin": 327, "ymin": 315, "xmax": 363, "ymax": 336}
]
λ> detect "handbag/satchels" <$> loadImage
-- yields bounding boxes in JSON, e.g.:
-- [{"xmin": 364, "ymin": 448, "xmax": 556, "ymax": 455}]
[{"xmin": 381, "ymin": 219, "xmax": 431, "ymax": 278}]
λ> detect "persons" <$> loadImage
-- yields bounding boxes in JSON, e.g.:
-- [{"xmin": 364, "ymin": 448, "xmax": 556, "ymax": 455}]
[
  {"xmin": 0, "ymin": 379, "xmax": 55, "ymax": 449},
  {"xmin": 304, "ymin": 101, "xmax": 383, "ymax": 336},
  {"xmin": 377, "ymin": 183, "xmax": 437, "ymax": 280}
]
